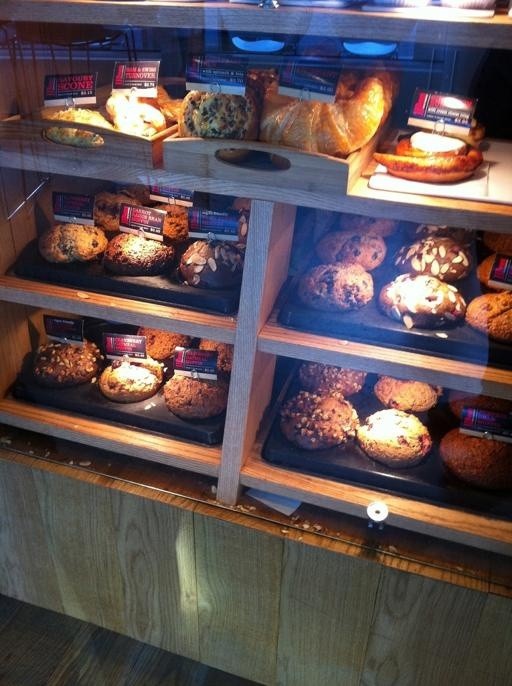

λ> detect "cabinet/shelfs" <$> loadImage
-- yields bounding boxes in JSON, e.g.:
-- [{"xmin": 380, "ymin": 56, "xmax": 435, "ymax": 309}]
[{"xmin": 0, "ymin": 1, "xmax": 512, "ymax": 686}]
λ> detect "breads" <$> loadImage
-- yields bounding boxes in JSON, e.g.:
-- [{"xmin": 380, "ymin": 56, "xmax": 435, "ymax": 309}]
[
  {"xmin": 295, "ymin": 212, "xmax": 512, "ymax": 344},
  {"xmin": 279, "ymin": 361, "xmax": 510, "ymax": 490},
  {"xmin": 32, "ymin": 327, "xmax": 234, "ymax": 421},
  {"xmin": 44, "ymin": 71, "xmax": 395, "ymax": 172},
  {"xmin": 372, "ymin": 131, "xmax": 484, "ymax": 183},
  {"xmin": 417, "ymin": 122, "xmax": 486, "ymax": 148},
  {"xmin": 35, "ymin": 190, "xmax": 251, "ymax": 291}
]
